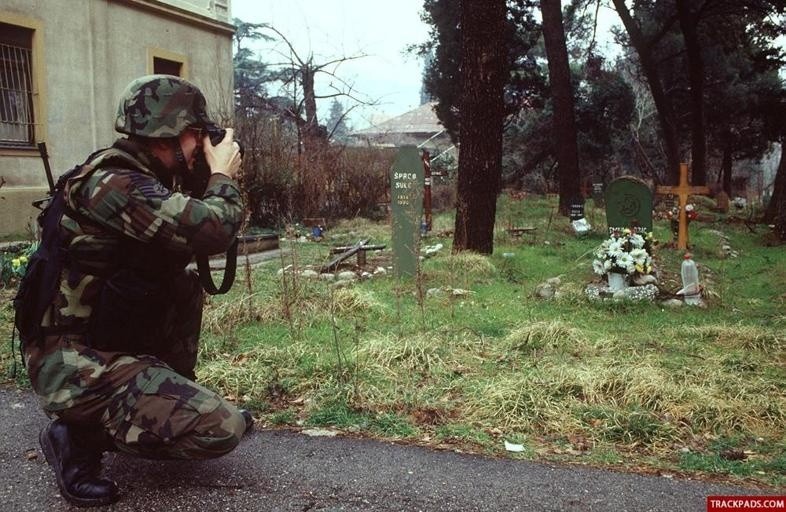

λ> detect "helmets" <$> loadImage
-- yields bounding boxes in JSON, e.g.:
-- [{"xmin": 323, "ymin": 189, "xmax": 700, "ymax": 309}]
[{"xmin": 115, "ymin": 74, "xmax": 215, "ymax": 138}]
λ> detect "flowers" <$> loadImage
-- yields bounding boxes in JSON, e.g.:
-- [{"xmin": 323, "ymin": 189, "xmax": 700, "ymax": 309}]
[{"xmin": 592, "ymin": 226, "xmax": 659, "ymax": 278}]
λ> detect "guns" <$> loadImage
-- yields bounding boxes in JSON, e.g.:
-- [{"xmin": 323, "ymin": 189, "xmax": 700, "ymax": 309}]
[{"xmin": 32, "ymin": 142, "xmax": 74, "ymax": 228}]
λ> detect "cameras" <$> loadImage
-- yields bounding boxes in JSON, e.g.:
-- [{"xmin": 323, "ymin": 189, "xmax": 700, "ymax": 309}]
[{"xmin": 201, "ymin": 133, "xmax": 244, "ymax": 174}]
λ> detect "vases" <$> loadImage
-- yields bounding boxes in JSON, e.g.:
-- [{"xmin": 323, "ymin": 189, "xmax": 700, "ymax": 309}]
[{"xmin": 607, "ymin": 272, "xmax": 630, "ymax": 294}]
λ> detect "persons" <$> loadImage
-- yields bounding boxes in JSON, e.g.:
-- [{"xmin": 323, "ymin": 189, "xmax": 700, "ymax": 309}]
[{"xmin": 10, "ymin": 73, "xmax": 248, "ymax": 507}]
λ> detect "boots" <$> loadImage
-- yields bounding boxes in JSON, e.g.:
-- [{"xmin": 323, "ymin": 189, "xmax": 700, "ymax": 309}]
[{"xmin": 39, "ymin": 417, "xmax": 121, "ymax": 506}]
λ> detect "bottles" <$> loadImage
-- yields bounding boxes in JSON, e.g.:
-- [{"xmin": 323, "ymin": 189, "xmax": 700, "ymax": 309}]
[
  {"xmin": 421, "ymin": 218, "xmax": 427, "ymax": 238},
  {"xmin": 681, "ymin": 254, "xmax": 701, "ymax": 305}
]
